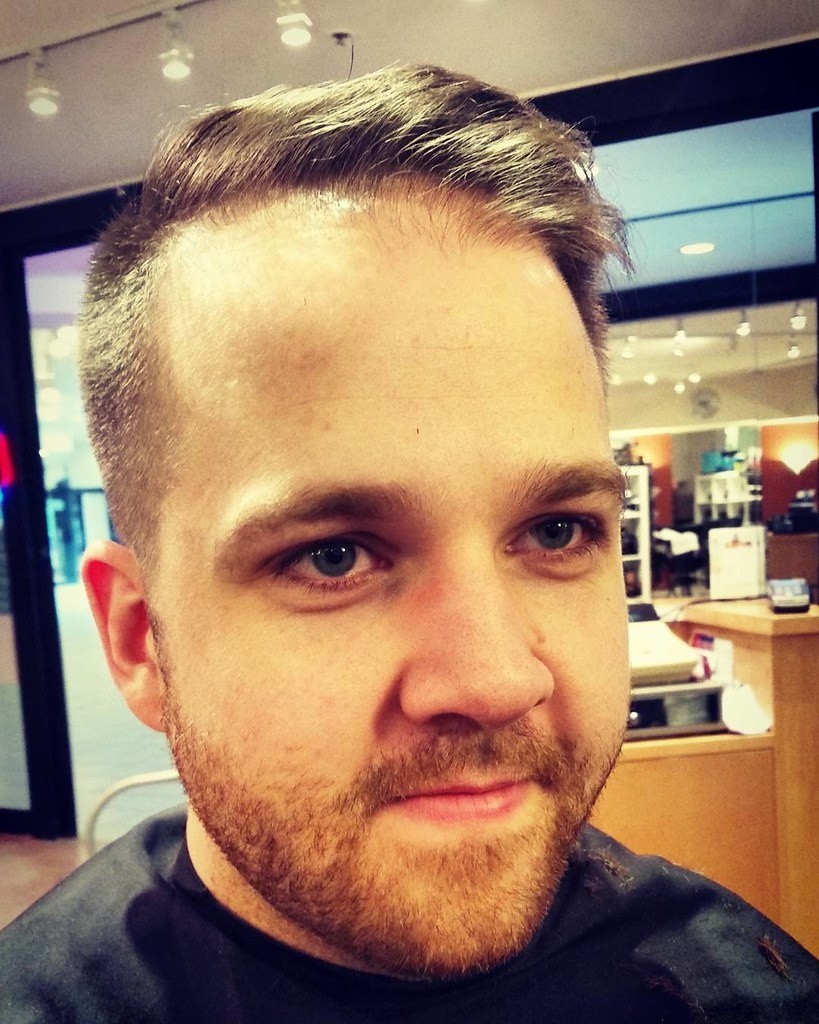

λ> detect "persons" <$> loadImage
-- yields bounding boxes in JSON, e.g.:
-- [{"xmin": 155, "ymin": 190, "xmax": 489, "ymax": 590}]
[{"xmin": 0, "ymin": 63, "xmax": 819, "ymax": 1024}]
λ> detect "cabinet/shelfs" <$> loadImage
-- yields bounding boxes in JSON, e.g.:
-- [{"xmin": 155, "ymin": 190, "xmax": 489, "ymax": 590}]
[{"xmin": 620, "ymin": 466, "xmax": 761, "ymax": 622}]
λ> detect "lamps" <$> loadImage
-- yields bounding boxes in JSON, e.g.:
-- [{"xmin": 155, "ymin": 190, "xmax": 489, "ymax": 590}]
[
  {"xmin": 25, "ymin": 46, "xmax": 61, "ymax": 115},
  {"xmin": 160, "ymin": 9, "xmax": 198, "ymax": 79},
  {"xmin": 276, "ymin": 0, "xmax": 312, "ymax": 44}
]
